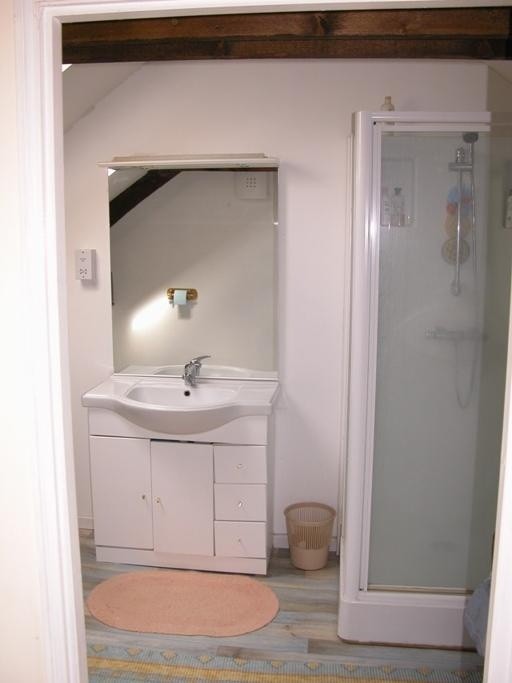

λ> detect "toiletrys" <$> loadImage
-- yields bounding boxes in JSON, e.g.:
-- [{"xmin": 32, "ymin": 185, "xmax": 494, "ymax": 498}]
[
  {"xmin": 380, "ymin": 188, "xmax": 391, "ymax": 226},
  {"xmin": 391, "ymin": 186, "xmax": 405, "ymax": 227}
]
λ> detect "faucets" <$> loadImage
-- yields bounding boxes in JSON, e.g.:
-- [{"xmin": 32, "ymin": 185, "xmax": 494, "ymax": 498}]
[
  {"xmin": 189, "ymin": 355, "xmax": 212, "ymax": 375},
  {"xmin": 184, "ymin": 363, "xmax": 196, "ymax": 386}
]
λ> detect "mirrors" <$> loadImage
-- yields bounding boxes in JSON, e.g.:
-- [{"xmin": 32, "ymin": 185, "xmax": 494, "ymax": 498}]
[{"xmin": 105, "ymin": 168, "xmax": 276, "ymax": 371}]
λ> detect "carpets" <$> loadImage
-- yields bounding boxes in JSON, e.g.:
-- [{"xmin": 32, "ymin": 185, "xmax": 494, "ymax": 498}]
[{"xmin": 87, "ymin": 573, "xmax": 281, "ymax": 639}]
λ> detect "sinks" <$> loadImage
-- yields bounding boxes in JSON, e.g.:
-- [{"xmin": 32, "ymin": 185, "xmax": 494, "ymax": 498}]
[
  {"xmin": 124, "ymin": 384, "xmax": 240, "ymax": 435},
  {"xmin": 148, "ymin": 365, "xmax": 243, "ymax": 379}
]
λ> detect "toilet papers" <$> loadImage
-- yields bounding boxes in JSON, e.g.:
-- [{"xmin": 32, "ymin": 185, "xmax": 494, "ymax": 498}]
[{"xmin": 173, "ymin": 289, "xmax": 188, "ymax": 307}]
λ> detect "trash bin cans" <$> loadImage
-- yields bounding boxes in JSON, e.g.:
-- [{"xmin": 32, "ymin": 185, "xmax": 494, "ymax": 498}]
[{"xmin": 283, "ymin": 502, "xmax": 336, "ymax": 570}]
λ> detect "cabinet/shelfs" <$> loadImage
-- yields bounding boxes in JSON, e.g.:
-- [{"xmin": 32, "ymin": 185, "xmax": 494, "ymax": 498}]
[{"xmin": 88, "ymin": 406, "xmax": 274, "ymax": 576}]
